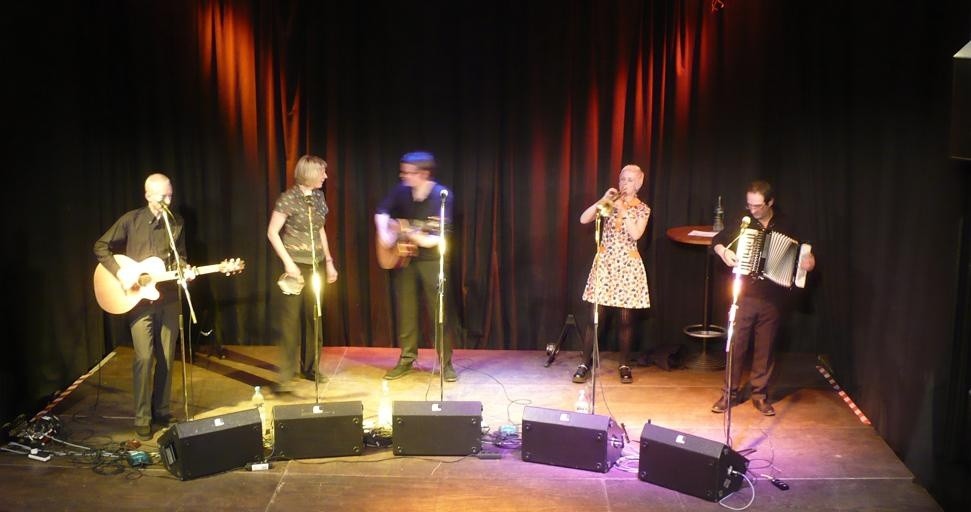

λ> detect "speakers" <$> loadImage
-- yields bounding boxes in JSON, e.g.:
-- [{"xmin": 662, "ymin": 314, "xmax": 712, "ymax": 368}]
[
  {"xmin": 272, "ymin": 401, "xmax": 364, "ymax": 459},
  {"xmin": 391, "ymin": 399, "xmax": 482, "ymax": 457},
  {"xmin": 521, "ymin": 406, "xmax": 625, "ymax": 473}
]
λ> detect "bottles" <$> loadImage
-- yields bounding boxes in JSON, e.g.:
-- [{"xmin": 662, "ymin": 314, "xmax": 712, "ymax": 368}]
[
  {"xmin": 252, "ymin": 386, "xmax": 267, "ymax": 436},
  {"xmin": 577, "ymin": 390, "xmax": 589, "ymax": 414},
  {"xmin": 713, "ymin": 197, "xmax": 726, "ymax": 231}
]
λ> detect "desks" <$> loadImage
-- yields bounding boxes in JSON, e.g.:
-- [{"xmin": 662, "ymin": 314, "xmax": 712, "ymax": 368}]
[{"xmin": 664, "ymin": 225, "xmax": 731, "ymax": 373}]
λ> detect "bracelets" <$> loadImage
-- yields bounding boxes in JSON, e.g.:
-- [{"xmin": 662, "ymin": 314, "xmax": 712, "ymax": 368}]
[{"xmin": 326, "ymin": 259, "xmax": 333, "ymax": 264}]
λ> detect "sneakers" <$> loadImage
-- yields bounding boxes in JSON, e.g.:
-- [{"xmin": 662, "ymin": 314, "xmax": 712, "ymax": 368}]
[
  {"xmin": 133, "ymin": 424, "xmax": 154, "ymax": 442},
  {"xmin": 150, "ymin": 413, "xmax": 179, "ymax": 425},
  {"xmin": 442, "ymin": 362, "xmax": 460, "ymax": 383},
  {"xmin": 382, "ymin": 362, "xmax": 418, "ymax": 380},
  {"xmin": 307, "ymin": 369, "xmax": 331, "ymax": 384}
]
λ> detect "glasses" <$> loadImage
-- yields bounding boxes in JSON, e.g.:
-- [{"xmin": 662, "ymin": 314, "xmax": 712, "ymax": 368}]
[{"xmin": 746, "ymin": 199, "xmax": 769, "ymax": 210}]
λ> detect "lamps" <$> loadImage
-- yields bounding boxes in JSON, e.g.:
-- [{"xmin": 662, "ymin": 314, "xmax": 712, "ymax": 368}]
[
  {"xmin": 518, "ymin": 402, "xmax": 630, "ymax": 475},
  {"xmin": 388, "ymin": 397, "xmax": 488, "ymax": 459},
  {"xmin": 266, "ymin": 398, "xmax": 370, "ymax": 462}
]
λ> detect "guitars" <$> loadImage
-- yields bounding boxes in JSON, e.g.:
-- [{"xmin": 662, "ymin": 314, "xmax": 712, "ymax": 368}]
[
  {"xmin": 92, "ymin": 254, "xmax": 246, "ymax": 315},
  {"xmin": 375, "ymin": 218, "xmax": 418, "ymax": 269}
]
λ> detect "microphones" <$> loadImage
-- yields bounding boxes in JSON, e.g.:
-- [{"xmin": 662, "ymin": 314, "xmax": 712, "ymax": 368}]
[
  {"xmin": 304, "ymin": 190, "xmax": 312, "ymax": 208},
  {"xmin": 156, "ymin": 196, "xmax": 174, "ymax": 219},
  {"xmin": 738, "ymin": 215, "xmax": 750, "ymax": 232},
  {"xmin": 440, "ymin": 189, "xmax": 447, "ymax": 208}
]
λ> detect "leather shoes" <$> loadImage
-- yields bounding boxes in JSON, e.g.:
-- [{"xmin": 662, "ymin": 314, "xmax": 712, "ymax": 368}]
[
  {"xmin": 618, "ymin": 364, "xmax": 634, "ymax": 383},
  {"xmin": 571, "ymin": 362, "xmax": 593, "ymax": 383},
  {"xmin": 710, "ymin": 392, "xmax": 741, "ymax": 414},
  {"xmin": 751, "ymin": 396, "xmax": 779, "ymax": 418}
]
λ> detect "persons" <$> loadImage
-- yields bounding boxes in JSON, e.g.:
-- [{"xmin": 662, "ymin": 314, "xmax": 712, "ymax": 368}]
[
  {"xmin": 572, "ymin": 164, "xmax": 651, "ymax": 383},
  {"xmin": 93, "ymin": 173, "xmax": 195, "ymax": 442},
  {"xmin": 267, "ymin": 155, "xmax": 339, "ymax": 384},
  {"xmin": 712, "ymin": 179, "xmax": 816, "ymax": 417},
  {"xmin": 374, "ymin": 153, "xmax": 456, "ymax": 383}
]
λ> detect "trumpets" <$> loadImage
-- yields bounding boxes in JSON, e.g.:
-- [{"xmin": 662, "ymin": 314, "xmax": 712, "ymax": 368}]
[{"xmin": 598, "ymin": 186, "xmax": 625, "ymax": 218}]
